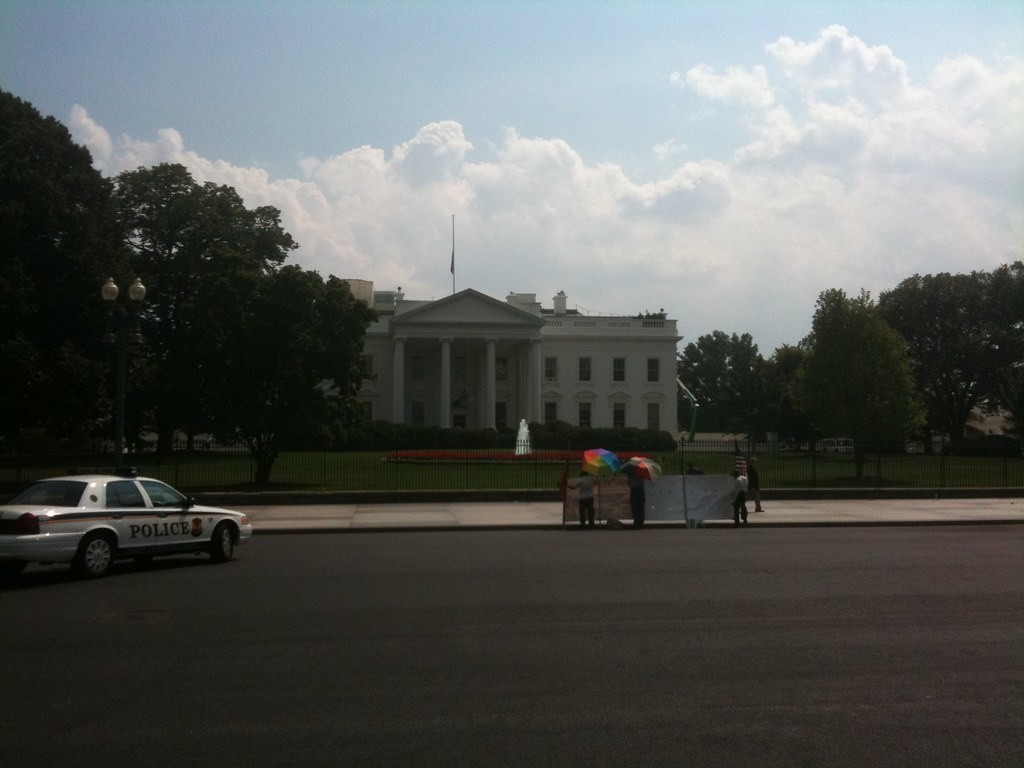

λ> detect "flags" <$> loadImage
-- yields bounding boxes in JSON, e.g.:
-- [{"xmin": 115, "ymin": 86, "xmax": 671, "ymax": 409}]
[
  {"xmin": 733, "ymin": 445, "xmax": 747, "ymax": 477},
  {"xmin": 450, "ymin": 224, "xmax": 455, "ymax": 275}
]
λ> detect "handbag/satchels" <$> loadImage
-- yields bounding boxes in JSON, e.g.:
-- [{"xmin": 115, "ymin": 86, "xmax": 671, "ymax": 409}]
[{"xmin": 736, "ymin": 491, "xmax": 746, "ymax": 506}]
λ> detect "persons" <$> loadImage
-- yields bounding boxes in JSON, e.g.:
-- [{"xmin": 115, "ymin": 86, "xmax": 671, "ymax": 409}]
[
  {"xmin": 517, "ymin": 418, "xmax": 530, "ymax": 438},
  {"xmin": 746, "ymin": 461, "xmax": 765, "ymax": 513},
  {"xmin": 731, "ymin": 469, "xmax": 750, "ymax": 528},
  {"xmin": 568, "ymin": 471, "xmax": 596, "ymax": 528},
  {"xmin": 624, "ymin": 472, "xmax": 646, "ymax": 528}
]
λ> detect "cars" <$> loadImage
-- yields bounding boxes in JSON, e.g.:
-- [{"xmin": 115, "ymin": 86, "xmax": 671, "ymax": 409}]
[{"xmin": 0, "ymin": 466, "xmax": 253, "ymax": 578}]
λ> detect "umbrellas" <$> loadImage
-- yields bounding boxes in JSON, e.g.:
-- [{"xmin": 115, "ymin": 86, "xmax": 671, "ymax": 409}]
[
  {"xmin": 615, "ymin": 456, "xmax": 662, "ymax": 481},
  {"xmin": 582, "ymin": 448, "xmax": 620, "ymax": 479}
]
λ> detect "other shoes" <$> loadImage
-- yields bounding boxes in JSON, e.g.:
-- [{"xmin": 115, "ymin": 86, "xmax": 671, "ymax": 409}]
[
  {"xmin": 731, "ymin": 523, "xmax": 739, "ymax": 527},
  {"xmin": 755, "ymin": 509, "xmax": 764, "ymax": 512},
  {"xmin": 630, "ymin": 522, "xmax": 644, "ymax": 529},
  {"xmin": 577, "ymin": 524, "xmax": 586, "ymax": 529},
  {"xmin": 587, "ymin": 523, "xmax": 595, "ymax": 529},
  {"xmin": 740, "ymin": 523, "xmax": 749, "ymax": 527}
]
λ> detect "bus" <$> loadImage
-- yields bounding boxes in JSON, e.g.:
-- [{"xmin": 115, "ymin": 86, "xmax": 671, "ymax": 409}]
[{"xmin": 815, "ymin": 438, "xmax": 855, "ymax": 453}]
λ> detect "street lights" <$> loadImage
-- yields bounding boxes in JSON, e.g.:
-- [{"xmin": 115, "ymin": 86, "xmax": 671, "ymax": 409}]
[{"xmin": 101, "ymin": 277, "xmax": 147, "ymax": 471}]
